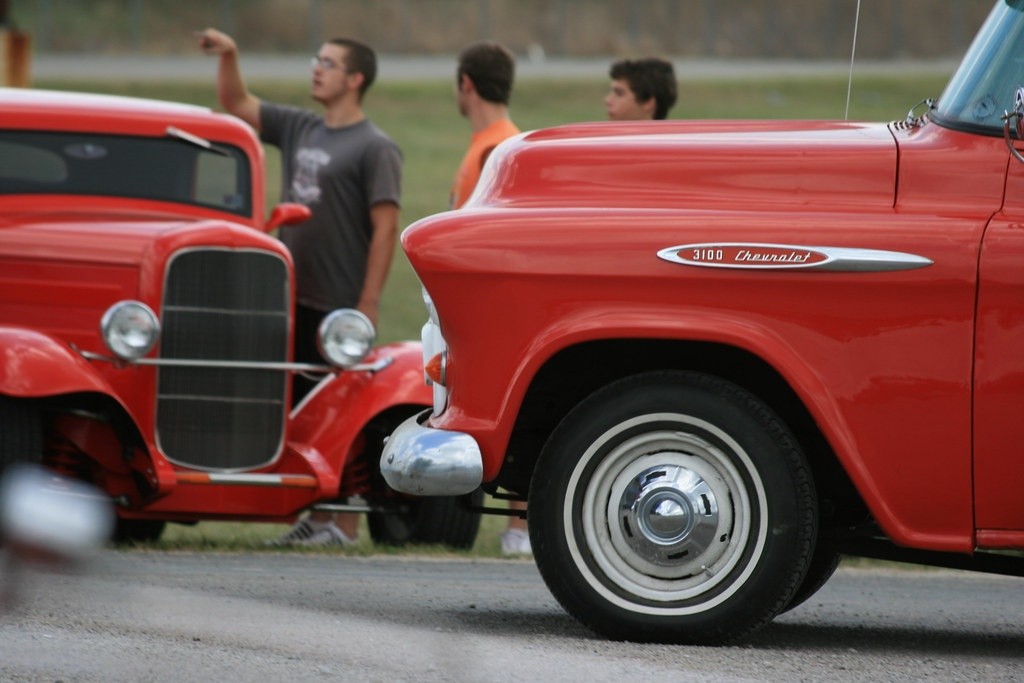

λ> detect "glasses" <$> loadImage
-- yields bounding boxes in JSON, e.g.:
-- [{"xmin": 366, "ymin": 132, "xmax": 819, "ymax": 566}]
[{"xmin": 312, "ymin": 55, "xmax": 341, "ymax": 70}]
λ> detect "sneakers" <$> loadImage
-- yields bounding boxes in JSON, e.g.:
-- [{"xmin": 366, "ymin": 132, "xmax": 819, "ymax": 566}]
[
  {"xmin": 291, "ymin": 522, "xmax": 359, "ymax": 550},
  {"xmin": 498, "ymin": 527, "xmax": 533, "ymax": 558},
  {"xmin": 265, "ymin": 519, "xmax": 333, "ymax": 547}
]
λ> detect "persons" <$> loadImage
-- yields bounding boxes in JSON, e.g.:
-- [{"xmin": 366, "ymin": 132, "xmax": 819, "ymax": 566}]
[
  {"xmin": 453, "ymin": 42, "xmax": 532, "ymax": 557},
  {"xmin": 195, "ymin": 29, "xmax": 404, "ymax": 549},
  {"xmin": 604, "ymin": 58, "xmax": 679, "ymax": 121}
]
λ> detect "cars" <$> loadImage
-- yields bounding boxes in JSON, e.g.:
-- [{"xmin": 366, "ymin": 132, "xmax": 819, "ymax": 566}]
[
  {"xmin": 377, "ymin": 0, "xmax": 1024, "ymax": 648},
  {"xmin": 2, "ymin": 84, "xmax": 481, "ymax": 552}
]
